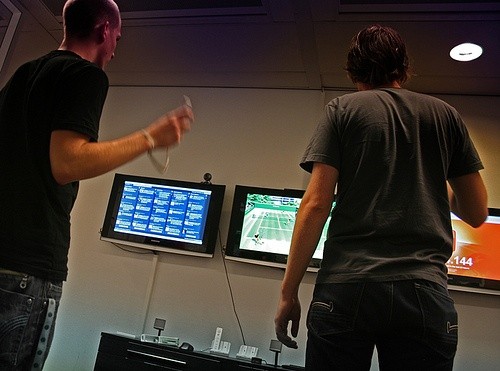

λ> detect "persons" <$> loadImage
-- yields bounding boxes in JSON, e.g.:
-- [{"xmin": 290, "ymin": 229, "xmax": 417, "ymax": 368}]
[
  {"xmin": 1, "ymin": 0, "xmax": 194, "ymax": 371},
  {"xmin": 274, "ymin": 24, "xmax": 489, "ymax": 371}
]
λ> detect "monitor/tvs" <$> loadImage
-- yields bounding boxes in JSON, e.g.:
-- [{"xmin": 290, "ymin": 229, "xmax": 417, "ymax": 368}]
[
  {"xmin": 224, "ymin": 185, "xmax": 337, "ymax": 273},
  {"xmin": 99, "ymin": 173, "xmax": 226, "ymax": 259},
  {"xmin": 445, "ymin": 208, "xmax": 500, "ymax": 296}
]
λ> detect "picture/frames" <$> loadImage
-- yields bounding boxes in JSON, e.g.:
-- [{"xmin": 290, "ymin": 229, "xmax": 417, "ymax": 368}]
[{"xmin": 0, "ymin": 0, "xmax": 23, "ymax": 73}]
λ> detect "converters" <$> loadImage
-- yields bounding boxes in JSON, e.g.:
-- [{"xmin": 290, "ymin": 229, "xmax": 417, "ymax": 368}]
[{"xmin": 251, "ymin": 356, "xmax": 263, "ymax": 364}]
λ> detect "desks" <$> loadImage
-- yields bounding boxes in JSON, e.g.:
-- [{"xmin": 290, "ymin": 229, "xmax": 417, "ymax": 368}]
[{"xmin": 92, "ymin": 329, "xmax": 285, "ymax": 371}]
